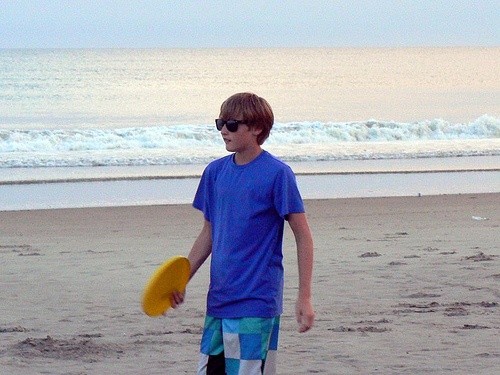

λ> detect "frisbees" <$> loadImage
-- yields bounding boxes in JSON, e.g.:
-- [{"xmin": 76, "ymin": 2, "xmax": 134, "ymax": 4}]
[{"xmin": 139, "ymin": 255, "xmax": 192, "ymax": 318}]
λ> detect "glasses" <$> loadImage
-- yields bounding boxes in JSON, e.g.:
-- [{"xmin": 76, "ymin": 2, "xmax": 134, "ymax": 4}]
[{"xmin": 214, "ymin": 118, "xmax": 254, "ymax": 133}]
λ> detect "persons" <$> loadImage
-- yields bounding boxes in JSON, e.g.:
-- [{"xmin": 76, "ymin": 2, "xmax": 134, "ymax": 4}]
[{"xmin": 161, "ymin": 93, "xmax": 314, "ymax": 375}]
